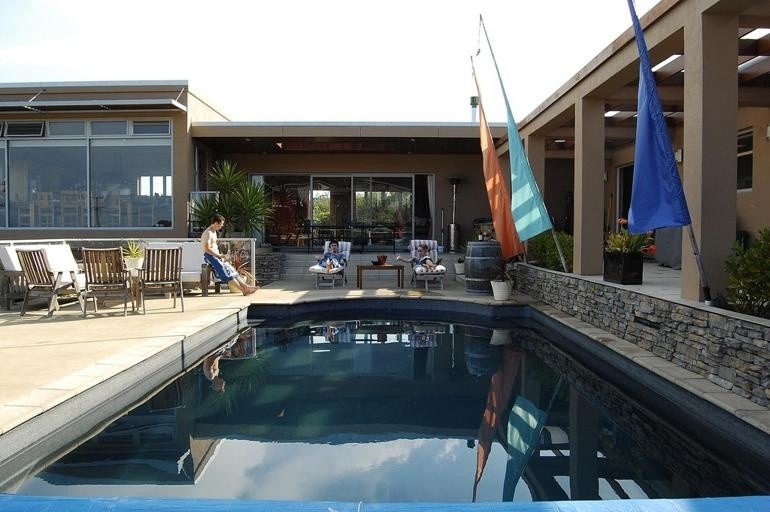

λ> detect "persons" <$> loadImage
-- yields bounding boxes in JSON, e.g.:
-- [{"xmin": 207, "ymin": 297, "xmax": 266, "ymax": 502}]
[
  {"xmin": 479, "ymin": 228, "xmax": 497, "ymax": 242},
  {"xmin": 318, "ymin": 239, "xmax": 347, "ymax": 271},
  {"xmin": 201, "ymin": 328, "xmax": 256, "ymax": 394},
  {"xmin": 396, "ymin": 244, "xmax": 442, "ymax": 272},
  {"xmin": 201, "ymin": 214, "xmax": 260, "ymax": 296},
  {"xmin": 418, "ymin": 331, "xmax": 437, "ymax": 344},
  {"xmin": 319, "ymin": 322, "xmax": 346, "ymax": 345},
  {"xmin": 616, "ymin": 216, "xmax": 629, "ymax": 225}
]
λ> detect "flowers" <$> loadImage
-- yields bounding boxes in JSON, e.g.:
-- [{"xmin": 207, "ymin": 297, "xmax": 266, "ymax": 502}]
[{"xmin": 607, "ymin": 216, "xmax": 658, "ymax": 254}]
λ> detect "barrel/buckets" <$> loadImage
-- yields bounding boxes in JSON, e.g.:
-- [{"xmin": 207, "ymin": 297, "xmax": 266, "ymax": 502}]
[{"xmin": 465, "ymin": 242, "xmax": 503, "ymax": 295}]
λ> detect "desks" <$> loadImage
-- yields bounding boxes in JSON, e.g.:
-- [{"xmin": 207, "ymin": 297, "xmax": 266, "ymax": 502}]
[{"xmin": 357, "ymin": 263, "xmax": 405, "ymax": 289}]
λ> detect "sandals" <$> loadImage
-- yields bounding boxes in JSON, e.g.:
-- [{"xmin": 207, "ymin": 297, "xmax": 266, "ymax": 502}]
[{"xmin": 243, "ymin": 286, "xmax": 260, "ymax": 296}]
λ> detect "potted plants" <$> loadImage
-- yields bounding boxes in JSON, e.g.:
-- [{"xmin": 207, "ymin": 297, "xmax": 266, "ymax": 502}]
[
  {"xmin": 486, "ymin": 248, "xmax": 527, "ymax": 300},
  {"xmin": 454, "ymin": 258, "xmax": 464, "ymax": 274}
]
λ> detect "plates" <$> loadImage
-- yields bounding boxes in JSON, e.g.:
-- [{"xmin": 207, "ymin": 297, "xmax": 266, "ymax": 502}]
[{"xmin": 372, "ymin": 261, "xmax": 381, "ymax": 265}]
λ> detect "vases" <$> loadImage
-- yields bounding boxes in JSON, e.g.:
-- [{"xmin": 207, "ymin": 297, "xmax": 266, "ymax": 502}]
[
  {"xmin": 490, "ymin": 329, "xmax": 515, "ymax": 347},
  {"xmin": 603, "ymin": 254, "xmax": 644, "ymax": 286}
]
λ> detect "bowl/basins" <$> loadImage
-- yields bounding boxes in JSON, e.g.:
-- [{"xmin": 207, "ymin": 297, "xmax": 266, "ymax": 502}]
[{"xmin": 377, "ymin": 255, "xmax": 387, "ymax": 265}]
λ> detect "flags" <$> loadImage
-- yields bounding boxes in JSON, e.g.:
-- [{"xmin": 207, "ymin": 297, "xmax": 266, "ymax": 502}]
[
  {"xmin": 626, "ymin": 0, "xmax": 692, "ymax": 234},
  {"xmin": 478, "ymin": 102, "xmax": 526, "ymax": 261},
  {"xmin": 507, "ymin": 106, "xmax": 556, "ymax": 242}
]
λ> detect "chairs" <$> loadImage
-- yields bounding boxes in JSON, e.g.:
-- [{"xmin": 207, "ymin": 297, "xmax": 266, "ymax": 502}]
[
  {"xmin": 272, "ymin": 228, "xmax": 393, "ymax": 246},
  {"xmin": 0, "ymin": 238, "xmax": 222, "ymax": 320},
  {"xmin": 310, "ymin": 241, "xmax": 351, "ymax": 288},
  {"xmin": 324, "ymin": 325, "xmax": 353, "ymax": 343},
  {"xmin": 410, "ymin": 239, "xmax": 446, "ymax": 290},
  {"xmin": 405, "ymin": 319, "xmax": 441, "ymax": 348}
]
